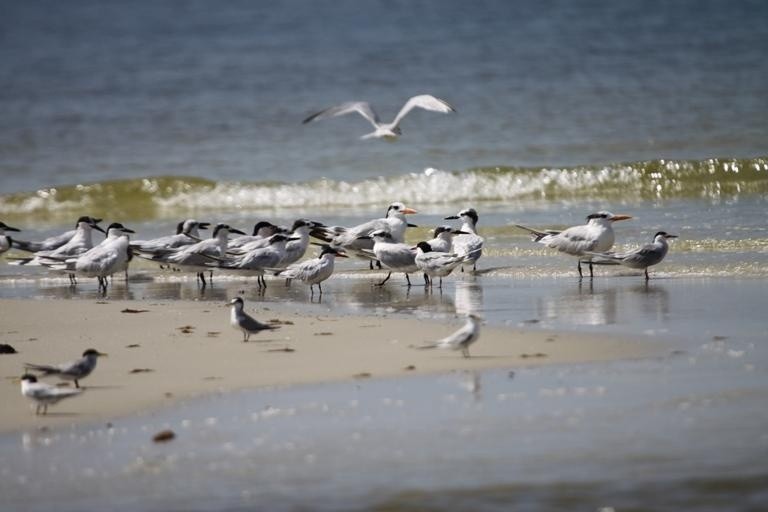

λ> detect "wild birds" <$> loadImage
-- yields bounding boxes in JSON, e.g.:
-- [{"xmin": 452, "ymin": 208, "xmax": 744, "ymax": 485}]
[
  {"xmin": 514, "ymin": 209, "xmax": 632, "ymax": 277},
  {"xmin": 0, "ymin": 201, "xmax": 486, "ymax": 294},
  {"xmin": 579, "ymin": 231, "xmax": 679, "ymax": 279},
  {"xmin": 23, "ymin": 349, "xmax": 108, "ymax": 389},
  {"xmin": 301, "ymin": 93, "xmax": 457, "ymax": 141},
  {"xmin": 11, "ymin": 374, "xmax": 83, "ymax": 416},
  {"xmin": 224, "ymin": 297, "xmax": 281, "ymax": 342},
  {"xmin": 416, "ymin": 315, "xmax": 483, "ymax": 358}
]
